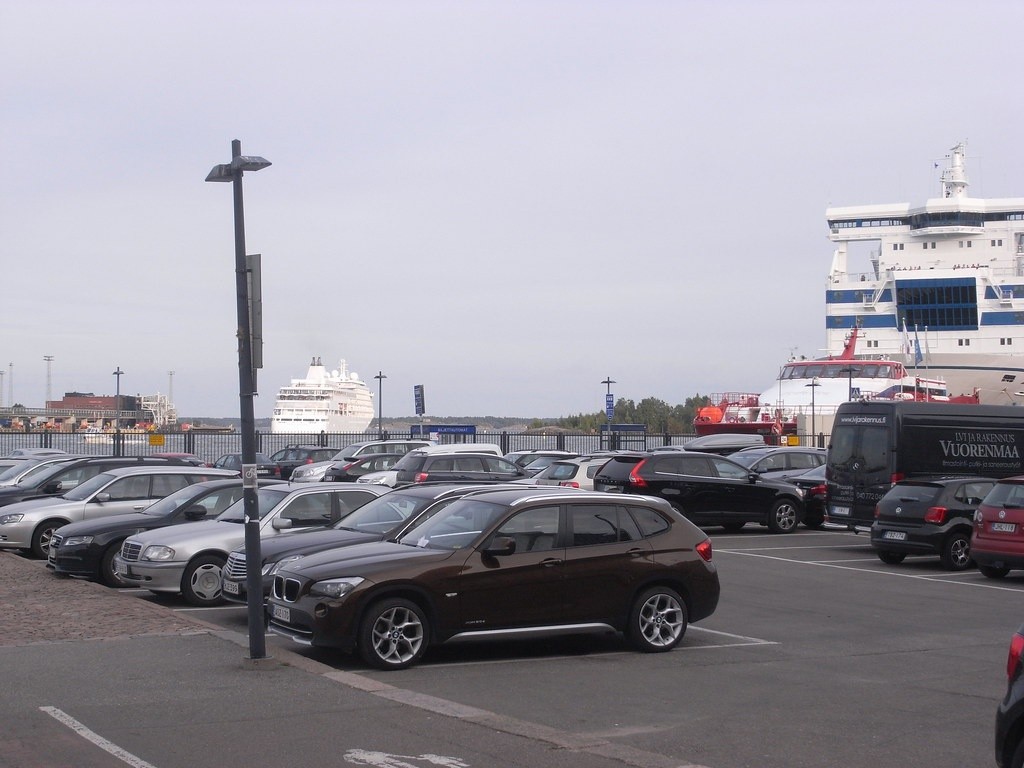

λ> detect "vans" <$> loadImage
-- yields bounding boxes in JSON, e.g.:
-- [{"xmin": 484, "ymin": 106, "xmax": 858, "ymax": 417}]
[{"xmin": 821, "ymin": 400, "xmax": 1023, "ymax": 534}]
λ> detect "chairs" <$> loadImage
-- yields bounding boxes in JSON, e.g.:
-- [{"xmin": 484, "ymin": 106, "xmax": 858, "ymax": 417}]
[{"xmin": 511, "ymin": 519, "xmax": 558, "ymax": 552}]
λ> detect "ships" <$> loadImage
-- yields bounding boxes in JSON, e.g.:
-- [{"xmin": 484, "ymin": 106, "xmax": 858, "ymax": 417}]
[
  {"xmin": 270, "ymin": 356, "xmax": 374, "ymax": 437},
  {"xmin": 821, "ymin": 139, "xmax": 1024, "ymax": 408}
]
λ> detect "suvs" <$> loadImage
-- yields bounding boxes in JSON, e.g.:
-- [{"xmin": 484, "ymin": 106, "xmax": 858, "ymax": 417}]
[
  {"xmin": 967, "ymin": 475, "xmax": 1024, "ymax": 579},
  {"xmin": 869, "ymin": 475, "xmax": 1024, "ymax": 571},
  {"xmin": 217, "ymin": 479, "xmax": 590, "ymax": 610},
  {"xmin": 716, "ymin": 446, "xmax": 829, "ymax": 480},
  {"xmin": 591, "ymin": 448, "xmax": 806, "ymax": 535},
  {"xmin": 266, "ymin": 485, "xmax": 721, "ymax": 671}
]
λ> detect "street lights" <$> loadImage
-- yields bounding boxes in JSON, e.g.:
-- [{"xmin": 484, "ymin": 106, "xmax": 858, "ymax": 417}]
[
  {"xmin": 804, "ymin": 378, "xmax": 823, "ymax": 447},
  {"xmin": 373, "ymin": 371, "xmax": 388, "ymax": 440},
  {"xmin": 601, "ymin": 376, "xmax": 617, "ymax": 450},
  {"xmin": 840, "ymin": 364, "xmax": 862, "ymax": 401},
  {"xmin": 112, "ymin": 366, "xmax": 125, "ymax": 456},
  {"xmin": 202, "ymin": 139, "xmax": 282, "ymax": 670}
]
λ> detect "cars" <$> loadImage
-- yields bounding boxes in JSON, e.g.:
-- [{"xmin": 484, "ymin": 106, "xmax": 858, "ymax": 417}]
[
  {"xmin": 0, "ymin": 432, "xmax": 767, "ymax": 607},
  {"xmin": 783, "ymin": 464, "xmax": 827, "ymax": 530}
]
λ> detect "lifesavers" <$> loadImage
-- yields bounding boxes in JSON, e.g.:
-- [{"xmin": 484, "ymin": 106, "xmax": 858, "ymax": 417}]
[
  {"xmin": 739, "ymin": 416, "xmax": 746, "ymax": 422},
  {"xmin": 340, "ymin": 404, "xmax": 343, "ymax": 410},
  {"xmin": 729, "ymin": 417, "xmax": 735, "ymax": 424},
  {"xmin": 771, "ymin": 423, "xmax": 782, "ymax": 434}
]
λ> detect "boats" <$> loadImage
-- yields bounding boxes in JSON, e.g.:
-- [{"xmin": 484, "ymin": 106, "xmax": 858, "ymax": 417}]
[{"xmin": 691, "ymin": 313, "xmax": 981, "ymax": 446}]
